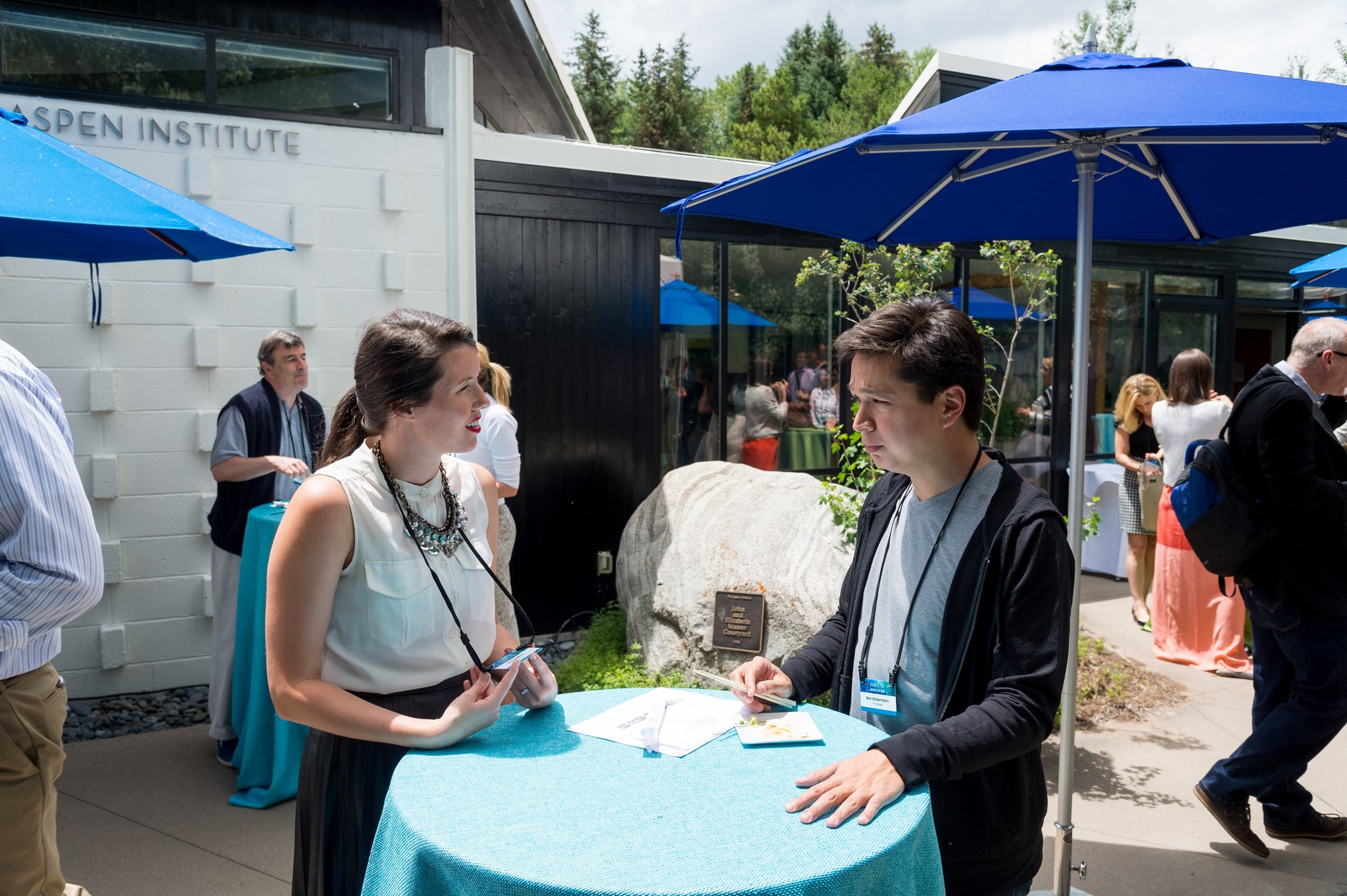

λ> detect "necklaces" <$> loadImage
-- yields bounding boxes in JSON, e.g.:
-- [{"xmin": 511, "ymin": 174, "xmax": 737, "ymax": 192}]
[{"xmin": 374, "ymin": 439, "xmax": 468, "ymax": 557}]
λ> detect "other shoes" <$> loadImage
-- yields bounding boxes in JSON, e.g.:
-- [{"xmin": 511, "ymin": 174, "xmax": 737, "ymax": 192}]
[
  {"xmin": 215, "ymin": 737, "xmax": 237, "ymax": 766},
  {"xmin": 1265, "ymin": 806, "xmax": 1347, "ymax": 840},
  {"xmin": 1193, "ymin": 782, "xmax": 1270, "ymax": 859},
  {"xmin": 1132, "ymin": 606, "xmax": 1149, "ymax": 625}
]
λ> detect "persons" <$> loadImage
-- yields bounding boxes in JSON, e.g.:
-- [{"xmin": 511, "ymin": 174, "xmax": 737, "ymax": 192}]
[
  {"xmin": 730, "ymin": 296, "xmax": 1078, "ymax": 896},
  {"xmin": 664, "ymin": 340, "xmax": 843, "ymax": 479},
  {"xmin": 1152, "ymin": 347, "xmax": 1254, "ymax": 680},
  {"xmin": 446, "ymin": 341, "xmax": 524, "ymax": 651},
  {"xmin": 206, "ymin": 330, "xmax": 327, "ymax": 766},
  {"xmin": 1113, "ymin": 374, "xmax": 1168, "ymax": 626},
  {"xmin": 1191, "ymin": 314, "xmax": 1347, "ymax": 860},
  {"xmin": 0, "ymin": 341, "xmax": 105, "ymax": 896},
  {"xmin": 981, "ymin": 355, "xmax": 1052, "ymax": 497},
  {"xmin": 264, "ymin": 308, "xmax": 558, "ymax": 896}
]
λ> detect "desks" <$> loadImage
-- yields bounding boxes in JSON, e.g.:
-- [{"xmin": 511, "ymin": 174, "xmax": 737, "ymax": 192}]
[
  {"xmin": 1066, "ymin": 463, "xmax": 1129, "ymax": 581},
  {"xmin": 361, "ymin": 688, "xmax": 946, "ymax": 895},
  {"xmin": 228, "ymin": 499, "xmax": 309, "ymax": 809},
  {"xmin": 775, "ymin": 427, "xmax": 840, "ymax": 481}
]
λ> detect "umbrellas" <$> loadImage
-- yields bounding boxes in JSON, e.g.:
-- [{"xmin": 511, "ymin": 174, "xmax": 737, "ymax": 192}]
[
  {"xmin": 943, "ymin": 284, "xmax": 1052, "ymax": 324},
  {"xmin": 661, "ymin": 23, "xmax": 1347, "ymax": 896},
  {"xmin": 1295, "ymin": 298, "xmax": 1347, "ymax": 324},
  {"xmin": 0, "ymin": 108, "xmax": 297, "ymax": 329},
  {"xmin": 1288, "ymin": 247, "xmax": 1347, "ymax": 293},
  {"xmin": 661, "ymin": 273, "xmax": 779, "ymax": 465}
]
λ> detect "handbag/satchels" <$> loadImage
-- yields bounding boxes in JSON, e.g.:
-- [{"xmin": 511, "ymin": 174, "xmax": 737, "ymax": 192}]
[{"xmin": 1137, "ymin": 455, "xmax": 1165, "ymax": 531}]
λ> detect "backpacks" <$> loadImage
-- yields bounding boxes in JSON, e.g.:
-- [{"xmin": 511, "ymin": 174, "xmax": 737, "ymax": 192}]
[{"xmin": 1171, "ymin": 376, "xmax": 1295, "ymax": 578}]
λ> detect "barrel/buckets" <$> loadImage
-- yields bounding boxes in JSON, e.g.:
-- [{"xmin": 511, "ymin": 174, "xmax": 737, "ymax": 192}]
[{"xmin": 1091, "ymin": 414, "xmax": 1116, "ymax": 454}]
